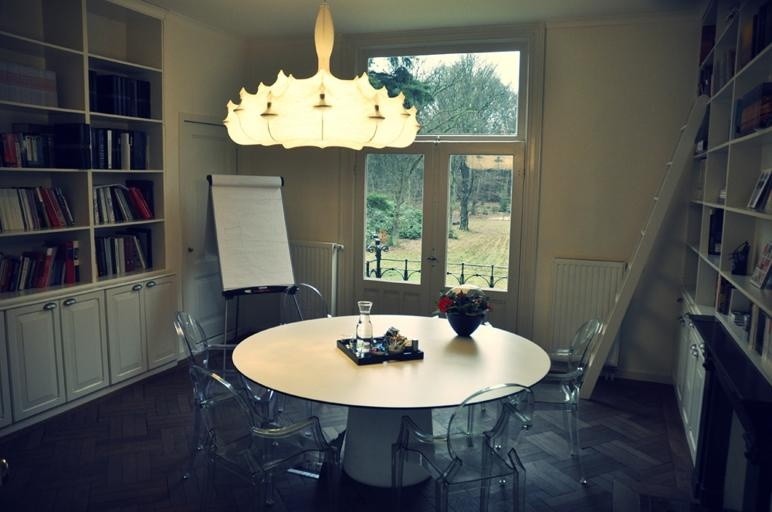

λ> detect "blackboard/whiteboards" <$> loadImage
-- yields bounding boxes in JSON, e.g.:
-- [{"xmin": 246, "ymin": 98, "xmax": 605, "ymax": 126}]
[{"xmin": 207, "ymin": 173, "xmax": 301, "ymax": 298}]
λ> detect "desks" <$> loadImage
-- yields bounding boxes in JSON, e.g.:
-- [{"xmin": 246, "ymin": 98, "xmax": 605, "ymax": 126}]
[{"xmin": 227, "ymin": 311, "xmax": 553, "ymax": 487}]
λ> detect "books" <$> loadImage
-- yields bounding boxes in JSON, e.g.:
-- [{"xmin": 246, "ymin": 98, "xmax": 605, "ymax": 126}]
[
  {"xmin": 1, "ymin": 47, "xmax": 156, "ymax": 295},
  {"xmin": 692, "ymin": 1, "xmax": 771, "ymax": 149}
]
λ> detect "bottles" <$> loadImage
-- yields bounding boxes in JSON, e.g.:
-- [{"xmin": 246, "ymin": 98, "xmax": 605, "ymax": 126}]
[{"xmin": 356, "ymin": 300, "xmax": 374, "ymax": 353}]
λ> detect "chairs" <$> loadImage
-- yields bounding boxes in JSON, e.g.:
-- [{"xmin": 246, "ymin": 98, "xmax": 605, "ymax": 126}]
[
  {"xmin": 171, "ymin": 310, "xmax": 287, "ymax": 461},
  {"xmin": 186, "ymin": 363, "xmax": 336, "ymax": 508},
  {"xmin": 434, "ymin": 282, "xmax": 492, "ymax": 327},
  {"xmin": 465, "ymin": 316, "xmax": 611, "ymax": 490},
  {"xmin": 279, "ymin": 281, "xmax": 336, "ymax": 417},
  {"xmin": 386, "ymin": 380, "xmax": 546, "ymax": 509}
]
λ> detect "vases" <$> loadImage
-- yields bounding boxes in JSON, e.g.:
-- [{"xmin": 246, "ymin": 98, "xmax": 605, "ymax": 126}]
[{"xmin": 445, "ymin": 309, "xmax": 489, "ymax": 337}]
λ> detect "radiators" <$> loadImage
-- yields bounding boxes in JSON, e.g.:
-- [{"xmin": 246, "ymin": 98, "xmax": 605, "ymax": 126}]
[
  {"xmin": 548, "ymin": 254, "xmax": 628, "ymax": 378},
  {"xmin": 281, "ymin": 237, "xmax": 346, "ymax": 324}
]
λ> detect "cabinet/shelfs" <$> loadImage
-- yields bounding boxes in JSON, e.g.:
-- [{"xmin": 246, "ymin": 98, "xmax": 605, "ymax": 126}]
[
  {"xmin": 4, "ymin": 286, "xmax": 111, "ymax": 427},
  {"xmin": 84, "ymin": 2, "xmax": 169, "ymax": 285},
  {"xmin": 1, "ymin": 0, "xmax": 96, "ymax": 297},
  {"xmin": 105, "ymin": 273, "xmax": 183, "ymax": 392}
]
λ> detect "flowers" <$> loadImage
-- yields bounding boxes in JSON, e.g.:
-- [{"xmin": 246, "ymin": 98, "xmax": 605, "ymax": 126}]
[{"xmin": 433, "ymin": 284, "xmax": 497, "ymax": 321}]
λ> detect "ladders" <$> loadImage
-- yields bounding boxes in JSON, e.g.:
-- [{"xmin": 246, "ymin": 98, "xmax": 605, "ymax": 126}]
[{"xmin": 578, "ymin": 93, "xmax": 709, "ymax": 400}]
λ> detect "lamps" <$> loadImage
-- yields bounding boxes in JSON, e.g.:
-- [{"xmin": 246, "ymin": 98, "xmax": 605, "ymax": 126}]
[{"xmin": 219, "ymin": 1, "xmax": 423, "ymax": 158}]
